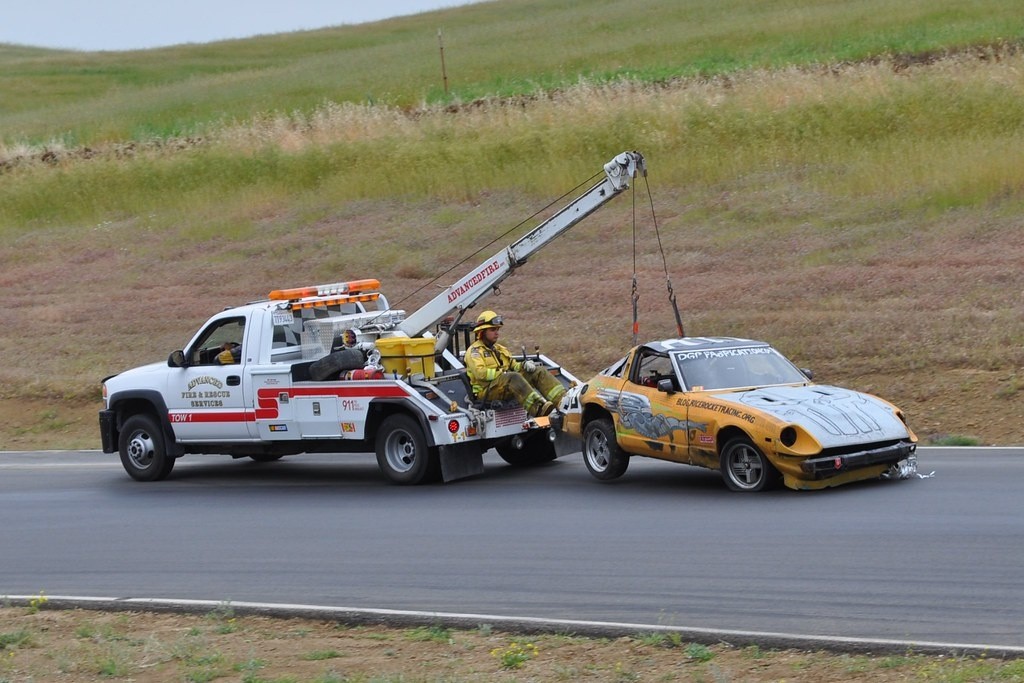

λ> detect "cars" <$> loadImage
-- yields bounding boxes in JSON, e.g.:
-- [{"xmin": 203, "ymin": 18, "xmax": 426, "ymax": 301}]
[{"xmin": 555, "ymin": 335, "xmax": 918, "ymax": 492}]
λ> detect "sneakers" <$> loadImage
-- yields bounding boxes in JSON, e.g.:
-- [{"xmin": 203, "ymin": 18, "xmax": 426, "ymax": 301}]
[{"xmin": 537, "ymin": 401, "xmax": 555, "ymax": 417}]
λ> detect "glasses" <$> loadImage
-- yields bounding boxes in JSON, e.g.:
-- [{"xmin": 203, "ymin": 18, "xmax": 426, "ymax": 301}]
[{"xmin": 484, "ymin": 316, "xmax": 504, "ymax": 326}]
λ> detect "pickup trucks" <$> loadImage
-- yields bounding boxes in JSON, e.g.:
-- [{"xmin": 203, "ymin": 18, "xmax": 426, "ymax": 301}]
[{"xmin": 99, "ymin": 277, "xmax": 583, "ymax": 484}]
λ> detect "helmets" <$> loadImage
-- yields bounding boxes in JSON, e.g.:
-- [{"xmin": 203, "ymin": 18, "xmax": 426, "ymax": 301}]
[{"xmin": 473, "ymin": 311, "xmax": 503, "ymax": 333}]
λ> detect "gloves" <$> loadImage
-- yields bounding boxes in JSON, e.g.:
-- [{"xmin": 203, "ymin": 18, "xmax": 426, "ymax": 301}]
[{"xmin": 524, "ymin": 360, "xmax": 536, "ymax": 372}]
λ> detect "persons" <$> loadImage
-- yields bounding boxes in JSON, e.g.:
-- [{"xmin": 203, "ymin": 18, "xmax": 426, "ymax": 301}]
[
  {"xmin": 464, "ymin": 311, "xmax": 577, "ymax": 417},
  {"xmin": 213, "ymin": 343, "xmax": 242, "ymax": 366}
]
